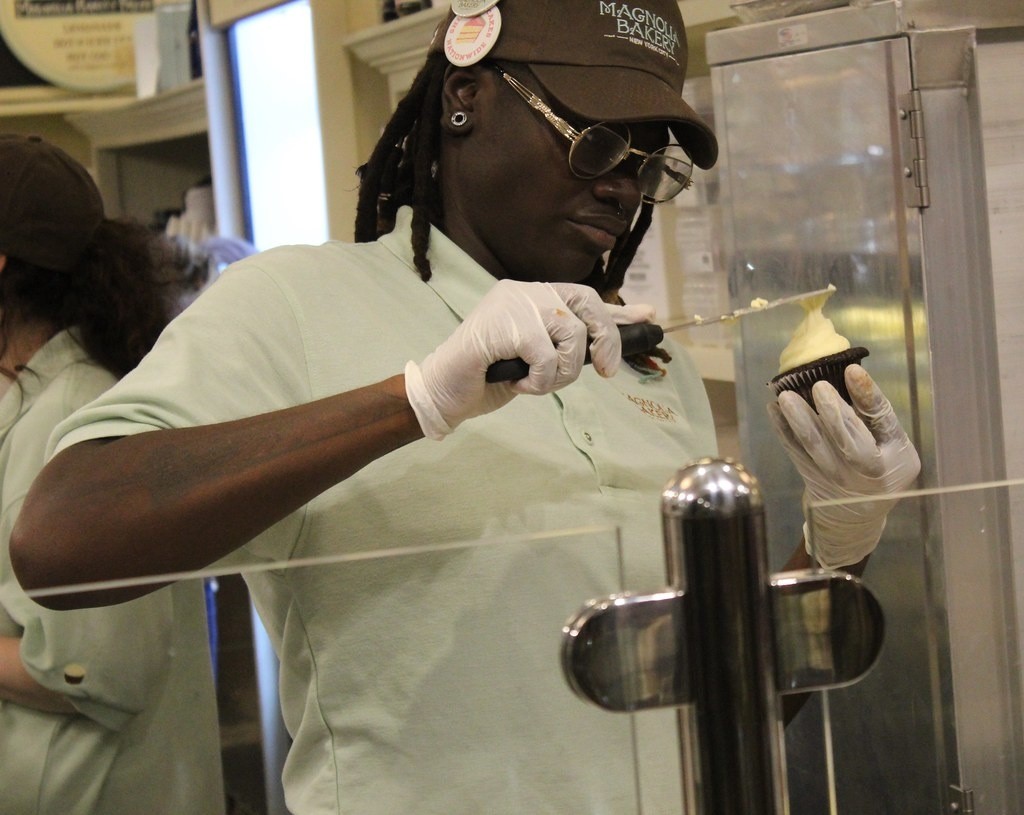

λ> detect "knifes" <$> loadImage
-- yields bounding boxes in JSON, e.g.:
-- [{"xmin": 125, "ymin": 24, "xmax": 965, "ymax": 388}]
[{"xmin": 484, "ymin": 287, "xmax": 836, "ymax": 383}]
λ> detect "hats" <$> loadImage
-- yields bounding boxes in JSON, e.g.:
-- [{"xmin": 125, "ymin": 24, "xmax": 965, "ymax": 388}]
[
  {"xmin": 425, "ymin": 0, "xmax": 718, "ymax": 170},
  {"xmin": 0, "ymin": 135, "xmax": 105, "ymax": 270}
]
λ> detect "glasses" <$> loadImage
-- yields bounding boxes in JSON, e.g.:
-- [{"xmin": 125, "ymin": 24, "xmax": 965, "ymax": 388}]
[{"xmin": 477, "ymin": 55, "xmax": 694, "ymax": 204}]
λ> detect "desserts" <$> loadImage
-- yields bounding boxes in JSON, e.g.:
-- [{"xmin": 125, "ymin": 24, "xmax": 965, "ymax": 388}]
[{"xmin": 768, "ymin": 294, "xmax": 869, "ymax": 415}]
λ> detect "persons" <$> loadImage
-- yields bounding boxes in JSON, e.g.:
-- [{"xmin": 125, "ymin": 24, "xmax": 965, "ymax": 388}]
[
  {"xmin": 0, "ymin": 130, "xmax": 226, "ymax": 815},
  {"xmin": 10, "ymin": 1, "xmax": 921, "ymax": 815}
]
